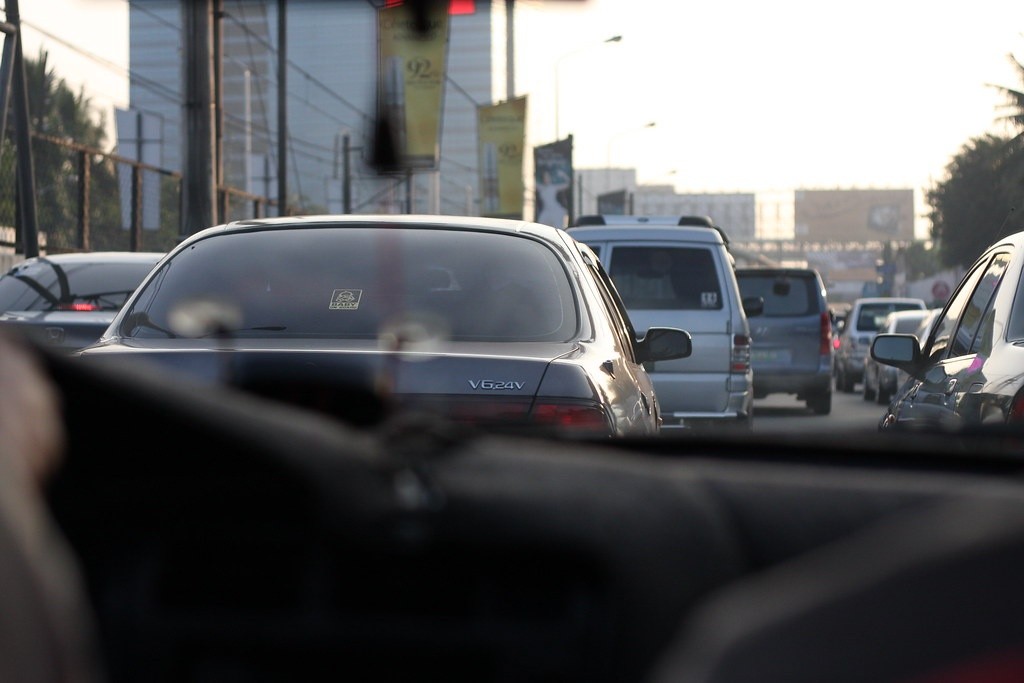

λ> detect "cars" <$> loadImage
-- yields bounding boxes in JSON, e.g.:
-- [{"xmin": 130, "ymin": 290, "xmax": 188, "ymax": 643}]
[
  {"xmin": 826, "ymin": 298, "xmax": 957, "ymax": 414},
  {"xmin": 0, "ymin": 252, "xmax": 171, "ymax": 375},
  {"xmin": 866, "ymin": 228, "xmax": 1023, "ymax": 441},
  {"xmin": 66, "ymin": 210, "xmax": 697, "ymax": 450}
]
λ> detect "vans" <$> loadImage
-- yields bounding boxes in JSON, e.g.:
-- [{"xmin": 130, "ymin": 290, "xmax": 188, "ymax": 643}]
[
  {"xmin": 729, "ymin": 264, "xmax": 847, "ymax": 413},
  {"xmin": 566, "ymin": 212, "xmax": 765, "ymax": 423}
]
